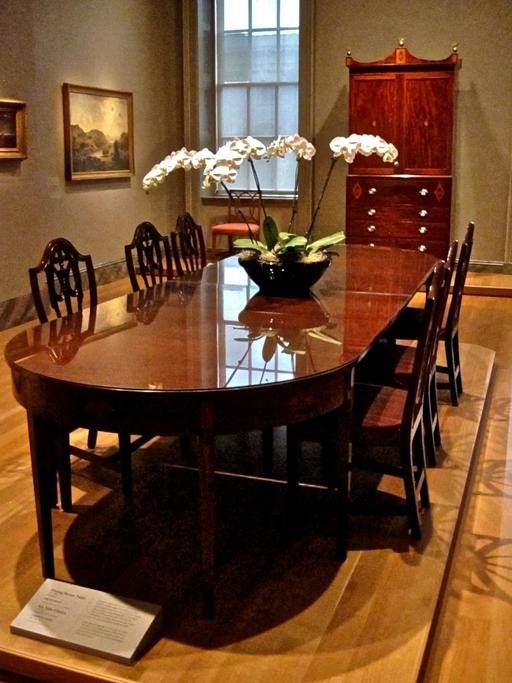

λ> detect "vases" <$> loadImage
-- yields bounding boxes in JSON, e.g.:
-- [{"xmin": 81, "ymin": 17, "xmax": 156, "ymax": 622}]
[
  {"xmin": 235, "ymin": 251, "xmax": 331, "ymax": 297},
  {"xmin": 237, "ymin": 288, "xmax": 331, "ymax": 328}
]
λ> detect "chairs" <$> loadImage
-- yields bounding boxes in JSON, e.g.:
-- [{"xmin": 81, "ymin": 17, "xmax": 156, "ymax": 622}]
[
  {"xmin": 211, "ymin": 189, "xmax": 263, "ymax": 263},
  {"xmin": 28, "ymin": 236, "xmax": 136, "ymax": 514},
  {"xmin": 353, "ymin": 238, "xmax": 461, "ymax": 467},
  {"xmin": 123, "ymin": 221, "xmax": 174, "ymax": 292},
  {"xmin": 170, "ymin": 211, "xmax": 207, "ymax": 277},
  {"xmin": 379, "ymin": 221, "xmax": 476, "ymax": 408},
  {"xmin": 286, "ymin": 260, "xmax": 448, "ymax": 542}
]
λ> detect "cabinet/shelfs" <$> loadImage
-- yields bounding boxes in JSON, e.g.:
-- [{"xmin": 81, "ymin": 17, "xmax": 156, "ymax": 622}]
[{"xmin": 345, "ymin": 39, "xmax": 463, "ymax": 260}]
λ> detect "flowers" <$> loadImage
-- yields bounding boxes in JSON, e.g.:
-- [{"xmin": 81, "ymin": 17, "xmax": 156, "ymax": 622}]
[
  {"xmin": 221, "ymin": 323, "xmax": 342, "ymax": 390},
  {"xmin": 142, "ymin": 130, "xmax": 399, "ymax": 263}
]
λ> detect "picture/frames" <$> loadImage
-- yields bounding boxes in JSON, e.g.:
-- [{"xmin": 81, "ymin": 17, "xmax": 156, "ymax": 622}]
[
  {"xmin": 0, "ymin": 96, "xmax": 27, "ymax": 159},
  {"xmin": 63, "ymin": 82, "xmax": 136, "ymax": 182}
]
